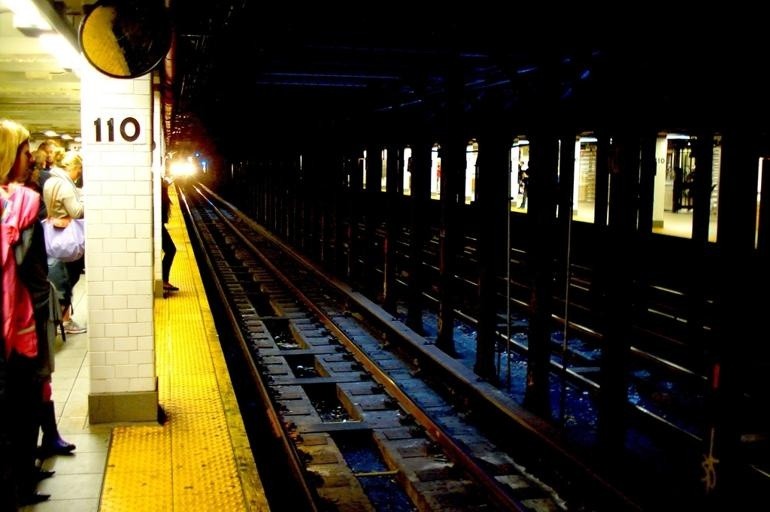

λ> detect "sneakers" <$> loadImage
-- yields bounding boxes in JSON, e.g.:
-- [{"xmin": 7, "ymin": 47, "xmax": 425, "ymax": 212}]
[{"xmin": 57, "ymin": 318, "xmax": 87, "ymax": 334}]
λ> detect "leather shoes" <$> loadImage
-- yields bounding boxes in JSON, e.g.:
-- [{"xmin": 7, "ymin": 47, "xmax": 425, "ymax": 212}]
[
  {"xmin": 36, "ymin": 466, "xmax": 55, "ymax": 480},
  {"xmin": 26, "ymin": 491, "xmax": 50, "ymax": 503},
  {"xmin": 163, "ymin": 284, "xmax": 179, "ymax": 290}
]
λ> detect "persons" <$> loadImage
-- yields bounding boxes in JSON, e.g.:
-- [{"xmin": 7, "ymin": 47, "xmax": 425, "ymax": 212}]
[
  {"xmin": 1, "ymin": 119, "xmax": 84, "ymax": 512},
  {"xmin": 161, "ymin": 178, "xmax": 182, "ymax": 294}
]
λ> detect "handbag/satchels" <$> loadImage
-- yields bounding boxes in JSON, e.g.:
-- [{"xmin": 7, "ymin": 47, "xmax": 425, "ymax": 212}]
[{"xmin": 41, "ymin": 216, "xmax": 84, "ymax": 263}]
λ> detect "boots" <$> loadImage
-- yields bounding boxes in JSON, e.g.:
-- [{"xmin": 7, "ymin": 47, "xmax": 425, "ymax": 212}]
[{"xmin": 37, "ymin": 401, "xmax": 75, "ymax": 458}]
